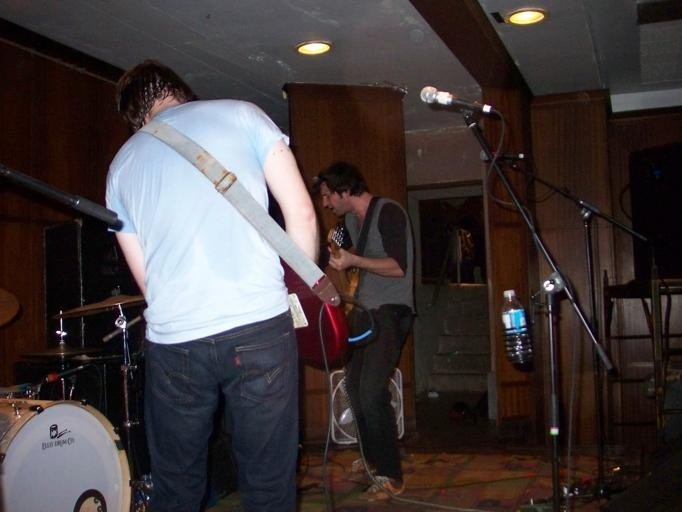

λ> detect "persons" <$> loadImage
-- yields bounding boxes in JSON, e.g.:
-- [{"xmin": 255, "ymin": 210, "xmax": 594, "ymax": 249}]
[
  {"xmin": 312, "ymin": 162, "xmax": 417, "ymax": 502},
  {"xmin": 107, "ymin": 58, "xmax": 323, "ymax": 512}
]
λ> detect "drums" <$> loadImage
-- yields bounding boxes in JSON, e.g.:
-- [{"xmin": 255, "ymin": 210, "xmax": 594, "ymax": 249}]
[{"xmin": 0, "ymin": 399, "xmax": 133, "ymax": 511}]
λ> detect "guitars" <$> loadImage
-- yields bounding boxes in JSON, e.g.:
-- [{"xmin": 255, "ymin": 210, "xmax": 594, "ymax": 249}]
[
  {"xmin": 280, "ymin": 257, "xmax": 348, "ymax": 367},
  {"xmin": 325, "ymin": 227, "xmax": 360, "ymax": 314}
]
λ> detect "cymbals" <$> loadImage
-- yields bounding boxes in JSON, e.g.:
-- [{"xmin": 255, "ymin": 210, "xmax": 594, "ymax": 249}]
[
  {"xmin": 56, "ymin": 296, "xmax": 143, "ymax": 318},
  {"xmin": 22, "ymin": 349, "xmax": 98, "ymax": 355}
]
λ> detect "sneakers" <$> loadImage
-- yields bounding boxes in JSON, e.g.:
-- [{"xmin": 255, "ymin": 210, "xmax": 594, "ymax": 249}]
[
  {"xmin": 354, "ymin": 466, "xmax": 378, "ymax": 482},
  {"xmin": 359, "ymin": 476, "xmax": 405, "ymax": 501}
]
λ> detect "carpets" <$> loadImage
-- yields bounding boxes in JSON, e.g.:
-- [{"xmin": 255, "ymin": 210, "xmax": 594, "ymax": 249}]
[{"xmin": 201, "ymin": 436, "xmax": 646, "ymax": 512}]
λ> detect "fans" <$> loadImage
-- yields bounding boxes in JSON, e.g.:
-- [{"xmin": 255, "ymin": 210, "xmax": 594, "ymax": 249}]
[{"xmin": 329, "ymin": 368, "xmax": 406, "ymax": 445}]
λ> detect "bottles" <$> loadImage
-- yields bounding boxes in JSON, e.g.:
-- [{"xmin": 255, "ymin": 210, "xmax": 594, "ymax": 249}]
[{"xmin": 501, "ymin": 288, "xmax": 533, "ymax": 366}]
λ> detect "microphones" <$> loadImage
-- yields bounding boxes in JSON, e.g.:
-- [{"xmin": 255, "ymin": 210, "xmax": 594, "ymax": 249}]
[
  {"xmin": 420, "ymin": 86, "xmax": 494, "ymax": 113},
  {"xmin": 480, "ymin": 150, "xmax": 526, "ymax": 162},
  {"xmin": 45, "ymin": 358, "xmax": 93, "ymax": 383},
  {"xmin": 101, "ymin": 312, "xmax": 145, "ymax": 343},
  {"xmin": 1, "ymin": 163, "xmax": 123, "ymax": 232}
]
